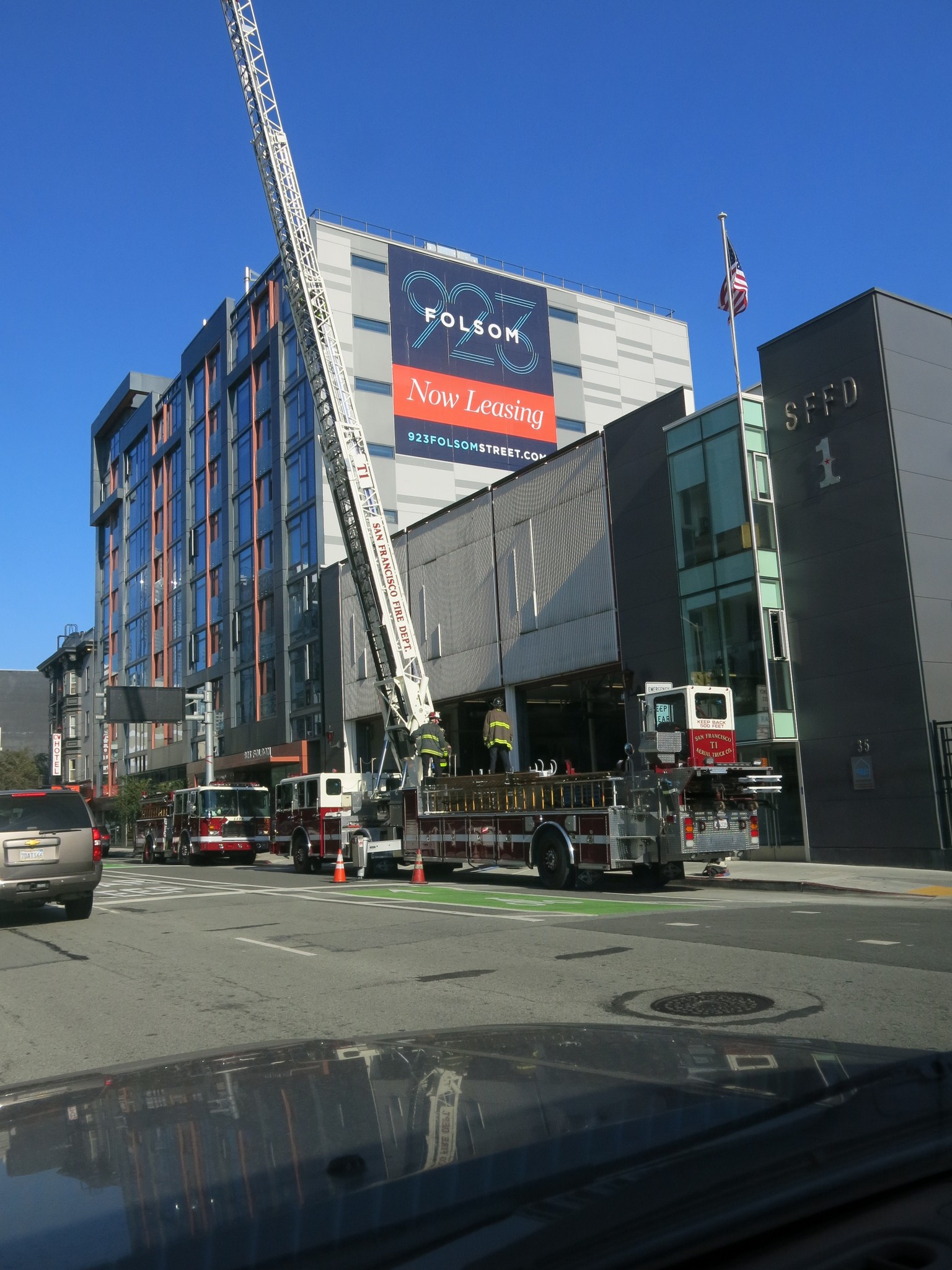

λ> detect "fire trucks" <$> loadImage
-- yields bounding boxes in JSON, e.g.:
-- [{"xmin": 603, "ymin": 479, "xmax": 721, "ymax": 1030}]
[
  {"xmin": 136, "ymin": 780, "xmax": 271, "ymax": 866},
  {"xmin": 220, "ymin": 0, "xmax": 785, "ymax": 891}
]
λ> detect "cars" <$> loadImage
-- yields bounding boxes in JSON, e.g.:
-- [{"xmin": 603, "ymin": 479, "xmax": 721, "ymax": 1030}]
[{"xmin": 97, "ymin": 825, "xmax": 110, "ymax": 857}]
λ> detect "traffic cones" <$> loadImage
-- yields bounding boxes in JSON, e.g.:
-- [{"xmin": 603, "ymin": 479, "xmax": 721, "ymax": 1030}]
[
  {"xmin": 329, "ymin": 849, "xmax": 350, "ymax": 883},
  {"xmin": 408, "ymin": 849, "xmax": 428, "ymax": 884}
]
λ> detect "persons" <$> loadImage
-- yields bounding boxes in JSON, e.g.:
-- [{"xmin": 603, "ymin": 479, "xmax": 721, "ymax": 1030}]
[
  {"xmin": 440, "ymin": 741, "xmax": 452, "ymax": 772},
  {"xmin": 409, "ymin": 712, "xmax": 445, "ymax": 777},
  {"xmin": 483, "ymin": 697, "xmax": 513, "ymax": 774}
]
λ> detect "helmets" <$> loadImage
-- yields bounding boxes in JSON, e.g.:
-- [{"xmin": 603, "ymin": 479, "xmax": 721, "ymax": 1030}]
[
  {"xmin": 428, "ymin": 712, "xmax": 442, "ymax": 721},
  {"xmin": 442, "ymin": 729, "xmax": 445, "ymax": 734},
  {"xmin": 491, "ymin": 697, "xmax": 504, "ymax": 707}
]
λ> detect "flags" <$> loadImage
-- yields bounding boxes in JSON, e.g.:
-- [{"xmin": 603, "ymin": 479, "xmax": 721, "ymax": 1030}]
[{"xmin": 718, "ymin": 234, "xmax": 748, "ymax": 325}]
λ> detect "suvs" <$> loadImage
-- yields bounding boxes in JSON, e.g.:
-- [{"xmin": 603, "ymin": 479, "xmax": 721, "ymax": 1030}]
[{"xmin": 0, "ymin": 784, "xmax": 103, "ymax": 920}]
